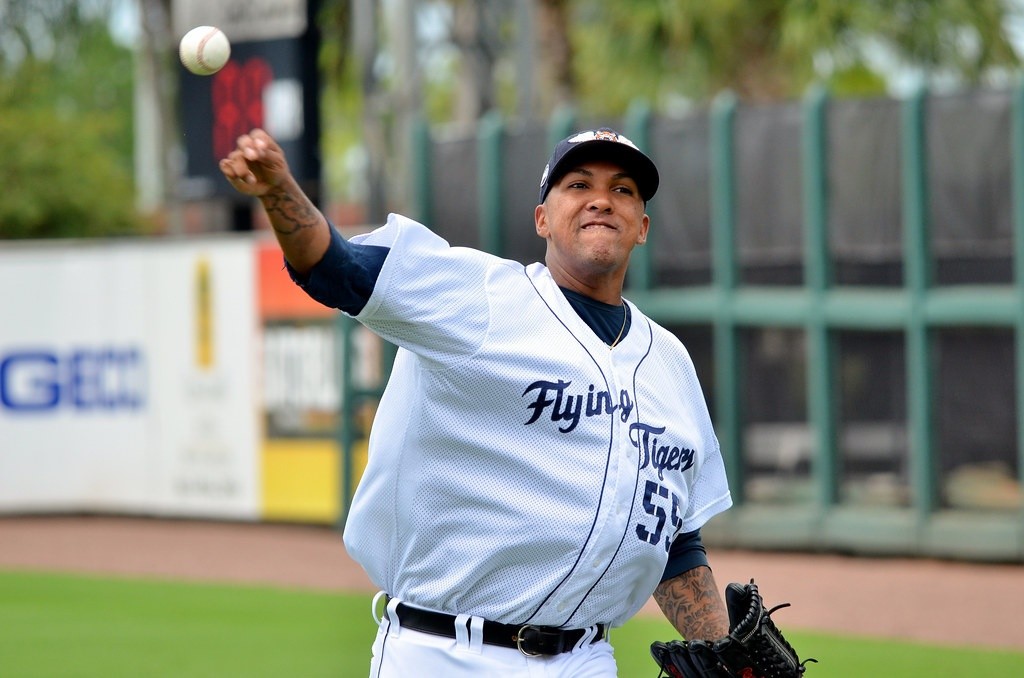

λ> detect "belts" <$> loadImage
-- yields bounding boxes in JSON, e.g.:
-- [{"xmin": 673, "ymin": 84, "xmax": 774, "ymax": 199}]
[{"xmin": 382, "ymin": 593, "xmax": 605, "ymax": 656}]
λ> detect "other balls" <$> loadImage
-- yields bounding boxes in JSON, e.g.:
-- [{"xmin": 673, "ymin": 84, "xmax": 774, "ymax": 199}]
[{"xmin": 178, "ymin": 24, "xmax": 232, "ymax": 78}]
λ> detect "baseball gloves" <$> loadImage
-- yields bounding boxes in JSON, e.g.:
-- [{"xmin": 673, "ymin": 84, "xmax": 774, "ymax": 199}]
[{"xmin": 647, "ymin": 573, "xmax": 820, "ymax": 678}]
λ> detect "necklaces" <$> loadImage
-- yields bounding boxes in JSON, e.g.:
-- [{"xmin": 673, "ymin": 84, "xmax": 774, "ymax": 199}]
[{"xmin": 607, "ymin": 301, "xmax": 626, "ymax": 349}]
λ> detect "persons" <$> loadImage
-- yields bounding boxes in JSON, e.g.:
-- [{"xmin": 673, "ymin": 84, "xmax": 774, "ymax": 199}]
[{"xmin": 218, "ymin": 127, "xmax": 732, "ymax": 678}]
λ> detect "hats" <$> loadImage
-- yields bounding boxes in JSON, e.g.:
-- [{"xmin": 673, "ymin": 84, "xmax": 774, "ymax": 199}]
[{"xmin": 540, "ymin": 128, "xmax": 660, "ymax": 205}]
